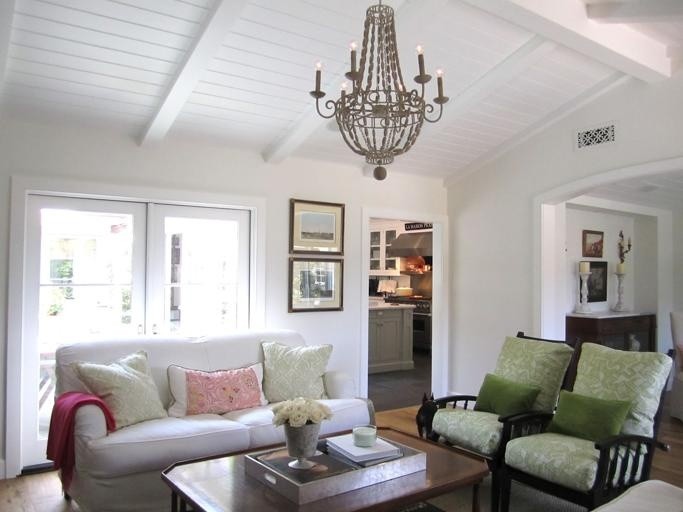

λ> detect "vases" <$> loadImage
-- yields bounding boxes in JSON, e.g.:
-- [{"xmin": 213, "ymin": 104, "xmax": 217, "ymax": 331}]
[{"xmin": 284, "ymin": 422, "xmax": 321, "ymax": 470}]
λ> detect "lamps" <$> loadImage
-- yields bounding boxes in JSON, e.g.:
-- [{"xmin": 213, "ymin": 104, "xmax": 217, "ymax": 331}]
[{"xmin": 309, "ymin": 0, "xmax": 450, "ymax": 180}]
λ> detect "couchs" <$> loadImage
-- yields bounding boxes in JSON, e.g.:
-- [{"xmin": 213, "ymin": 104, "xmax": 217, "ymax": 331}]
[{"xmin": 43, "ymin": 328, "xmax": 377, "ymax": 511}]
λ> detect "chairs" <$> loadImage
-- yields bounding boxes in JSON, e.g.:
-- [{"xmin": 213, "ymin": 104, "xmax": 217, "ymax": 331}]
[
  {"xmin": 424, "ymin": 331, "xmax": 580, "ymax": 511},
  {"xmin": 500, "ymin": 336, "xmax": 677, "ymax": 511}
]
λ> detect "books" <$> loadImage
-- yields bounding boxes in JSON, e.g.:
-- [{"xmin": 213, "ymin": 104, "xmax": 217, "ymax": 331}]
[{"xmin": 324, "ymin": 431, "xmax": 404, "ymax": 467}]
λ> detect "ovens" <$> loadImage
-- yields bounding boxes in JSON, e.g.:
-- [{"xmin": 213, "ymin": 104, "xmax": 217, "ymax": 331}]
[{"xmin": 412, "ymin": 316, "xmax": 430, "ymax": 352}]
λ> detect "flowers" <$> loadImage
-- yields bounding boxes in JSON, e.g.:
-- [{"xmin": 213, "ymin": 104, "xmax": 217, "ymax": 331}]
[{"xmin": 271, "ymin": 397, "xmax": 335, "ymax": 428}]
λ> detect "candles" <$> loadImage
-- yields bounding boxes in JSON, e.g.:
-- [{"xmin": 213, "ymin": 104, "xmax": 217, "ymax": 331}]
[
  {"xmin": 580, "ymin": 261, "xmax": 591, "ymax": 272},
  {"xmin": 616, "ymin": 262, "xmax": 624, "ymax": 273}
]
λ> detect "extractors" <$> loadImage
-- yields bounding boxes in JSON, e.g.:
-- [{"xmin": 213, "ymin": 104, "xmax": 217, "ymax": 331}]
[{"xmin": 388, "ymin": 231, "xmax": 432, "ymax": 257}]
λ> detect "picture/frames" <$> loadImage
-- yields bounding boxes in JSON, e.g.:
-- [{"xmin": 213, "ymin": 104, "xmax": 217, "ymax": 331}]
[
  {"xmin": 288, "ymin": 197, "xmax": 345, "ymax": 255},
  {"xmin": 581, "ymin": 230, "xmax": 604, "ymax": 258},
  {"xmin": 288, "ymin": 257, "xmax": 344, "ymax": 312},
  {"xmin": 579, "ymin": 261, "xmax": 608, "ymax": 303}
]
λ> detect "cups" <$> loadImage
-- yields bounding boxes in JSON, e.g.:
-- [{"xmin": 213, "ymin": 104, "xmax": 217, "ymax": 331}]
[{"xmin": 352, "ymin": 424, "xmax": 377, "ymax": 447}]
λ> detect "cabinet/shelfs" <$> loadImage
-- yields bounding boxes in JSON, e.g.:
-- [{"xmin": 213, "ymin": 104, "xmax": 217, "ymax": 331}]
[
  {"xmin": 564, "ymin": 310, "xmax": 657, "ymax": 353},
  {"xmin": 370, "ymin": 218, "xmax": 404, "ymax": 278}
]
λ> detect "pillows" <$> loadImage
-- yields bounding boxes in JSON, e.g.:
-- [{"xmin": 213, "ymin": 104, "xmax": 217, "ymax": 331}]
[
  {"xmin": 544, "ymin": 391, "xmax": 637, "ymax": 441},
  {"xmin": 71, "ymin": 351, "xmax": 169, "ymax": 433},
  {"xmin": 472, "ymin": 372, "xmax": 542, "ymax": 415},
  {"xmin": 166, "ymin": 361, "xmax": 271, "ymax": 419},
  {"xmin": 259, "ymin": 339, "xmax": 332, "ymax": 402}
]
